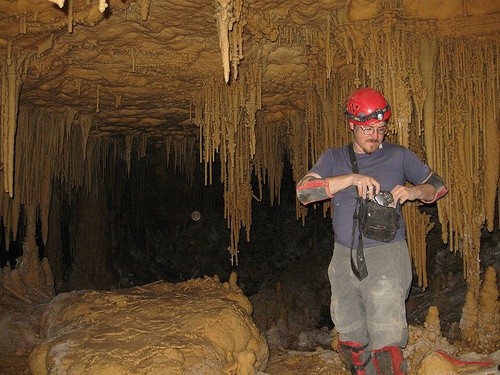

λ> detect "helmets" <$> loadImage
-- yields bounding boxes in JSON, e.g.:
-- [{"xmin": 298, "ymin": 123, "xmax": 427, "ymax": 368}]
[{"xmin": 344, "ymin": 89, "xmax": 392, "ymax": 126}]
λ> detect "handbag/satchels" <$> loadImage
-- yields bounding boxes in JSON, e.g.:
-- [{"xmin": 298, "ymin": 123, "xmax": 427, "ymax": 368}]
[{"xmin": 354, "ymin": 196, "xmax": 400, "ymax": 243}]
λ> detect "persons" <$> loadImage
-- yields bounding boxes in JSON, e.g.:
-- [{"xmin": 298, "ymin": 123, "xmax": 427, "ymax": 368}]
[{"xmin": 296, "ymin": 87, "xmax": 450, "ymax": 375}]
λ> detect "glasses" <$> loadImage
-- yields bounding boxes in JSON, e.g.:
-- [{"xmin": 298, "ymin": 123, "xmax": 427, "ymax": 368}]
[{"xmin": 359, "ymin": 125, "xmax": 388, "ymax": 135}]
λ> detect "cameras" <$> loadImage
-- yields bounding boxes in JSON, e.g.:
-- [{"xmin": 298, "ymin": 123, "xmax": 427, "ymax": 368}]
[{"xmin": 363, "ymin": 188, "xmax": 393, "ymax": 208}]
